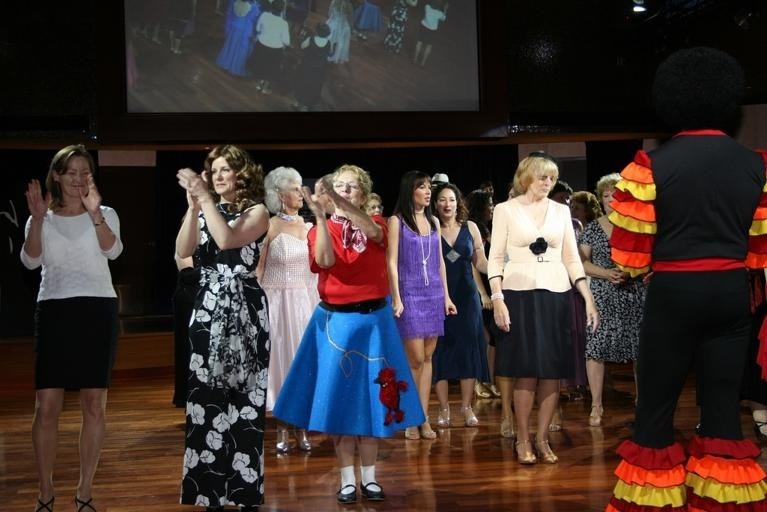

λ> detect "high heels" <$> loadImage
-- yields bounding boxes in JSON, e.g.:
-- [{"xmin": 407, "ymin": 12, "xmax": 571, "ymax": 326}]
[
  {"xmin": 460, "ymin": 406, "xmax": 479, "ymax": 428},
  {"xmin": 500, "ymin": 409, "xmax": 564, "ymax": 464},
  {"xmin": 560, "ymin": 385, "xmax": 586, "ymax": 402},
  {"xmin": 405, "ymin": 408, "xmax": 450, "ymax": 439},
  {"xmin": 294, "ymin": 429, "xmax": 312, "ymax": 451},
  {"xmin": 276, "ymin": 430, "xmax": 291, "ymax": 452},
  {"xmin": 589, "ymin": 405, "xmax": 604, "ymax": 427},
  {"xmin": 473, "ymin": 381, "xmax": 502, "ymax": 399}
]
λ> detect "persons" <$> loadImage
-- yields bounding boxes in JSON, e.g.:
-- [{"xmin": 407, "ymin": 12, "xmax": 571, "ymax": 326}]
[
  {"xmin": 630, "ymin": 142, "xmax": 649, "ymax": 511},
  {"xmin": 387, "ymin": 171, "xmax": 457, "ymax": 438},
  {"xmin": 271, "ymin": 166, "xmax": 426, "ymax": 503},
  {"xmin": 486, "ymin": 157, "xmax": 600, "ymax": 464},
  {"xmin": 255, "ymin": 167, "xmax": 319, "ymax": 453},
  {"xmin": 173, "ymin": 145, "xmax": 271, "ymax": 509},
  {"xmin": 214, "ymin": 0, "xmax": 449, "ymax": 104},
  {"xmin": 365, "ymin": 173, "xmax": 649, "ymax": 437},
  {"xmin": 605, "ymin": 45, "xmax": 766, "ymax": 512},
  {"xmin": 18, "ymin": 145, "xmax": 123, "ymax": 512}
]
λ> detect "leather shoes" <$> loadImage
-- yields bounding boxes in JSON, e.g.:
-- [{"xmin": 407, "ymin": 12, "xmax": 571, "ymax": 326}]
[
  {"xmin": 360, "ymin": 482, "xmax": 386, "ymax": 500},
  {"xmin": 753, "ymin": 420, "xmax": 766, "ymax": 444},
  {"xmin": 337, "ymin": 483, "xmax": 356, "ymax": 503}
]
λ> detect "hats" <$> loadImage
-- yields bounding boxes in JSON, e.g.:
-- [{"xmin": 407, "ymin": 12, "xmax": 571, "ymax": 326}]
[{"xmin": 432, "ymin": 173, "xmax": 449, "ymax": 187}]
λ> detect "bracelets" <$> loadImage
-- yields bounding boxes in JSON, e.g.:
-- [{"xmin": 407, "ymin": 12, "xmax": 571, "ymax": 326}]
[{"xmin": 92, "ymin": 216, "xmax": 106, "ymax": 227}]
